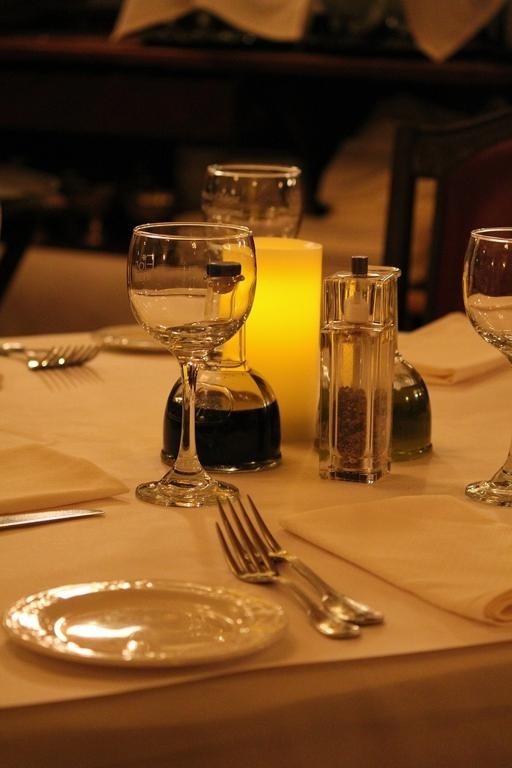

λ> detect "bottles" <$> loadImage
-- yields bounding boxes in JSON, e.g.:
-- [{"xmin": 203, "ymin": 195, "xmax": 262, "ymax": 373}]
[
  {"xmin": 312, "ymin": 350, "xmax": 435, "ymax": 461},
  {"xmin": 318, "ymin": 254, "xmax": 401, "ymax": 486},
  {"xmin": 161, "ymin": 259, "xmax": 283, "ymax": 475}
]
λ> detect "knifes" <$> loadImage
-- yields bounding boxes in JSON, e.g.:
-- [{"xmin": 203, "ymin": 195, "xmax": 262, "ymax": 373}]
[{"xmin": 0, "ymin": 506, "xmax": 105, "ymax": 532}]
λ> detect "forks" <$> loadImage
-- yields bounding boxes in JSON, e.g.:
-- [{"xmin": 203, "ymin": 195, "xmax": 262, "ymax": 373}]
[
  {"xmin": 2, "ymin": 341, "xmax": 105, "ymax": 372},
  {"xmin": 207, "ymin": 494, "xmax": 384, "ymax": 640}
]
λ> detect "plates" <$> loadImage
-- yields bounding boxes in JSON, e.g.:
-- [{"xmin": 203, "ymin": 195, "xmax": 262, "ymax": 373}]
[
  {"xmin": 3, "ymin": 578, "xmax": 290, "ymax": 670},
  {"xmin": 86, "ymin": 327, "xmax": 169, "ymax": 358}
]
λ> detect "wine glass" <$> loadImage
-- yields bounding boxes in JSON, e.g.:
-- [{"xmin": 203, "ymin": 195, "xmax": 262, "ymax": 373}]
[
  {"xmin": 462, "ymin": 223, "xmax": 512, "ymax": 509},
  {"xmin": 126, "ymin": 222, "xmax": 257, "ymax": 510}
]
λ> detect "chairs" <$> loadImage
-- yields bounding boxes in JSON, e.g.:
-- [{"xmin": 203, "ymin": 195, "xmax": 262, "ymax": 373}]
[
  {"xmin": 383, "ymin": 106, "xmax": 512, "ymax": 330},
  {"xmin": 2, "ymin": 245, "xmax": 208, "ymax": 337}
]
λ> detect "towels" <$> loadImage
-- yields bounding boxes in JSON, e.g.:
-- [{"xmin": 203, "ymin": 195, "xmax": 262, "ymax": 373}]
[
  {"xmin": 1, "ymin": 446, "xmax": 133, "ymax": 514},
  {"xmin": 396, "ymin": 311, "xmax": 509, "ymax": 385},
  {"xmin": 276, "ymin": 492, "xmax": 512, "ymax": 631}
]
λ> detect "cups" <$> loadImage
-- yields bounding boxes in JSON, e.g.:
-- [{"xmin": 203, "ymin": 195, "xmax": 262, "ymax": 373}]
[{"xmin": 200, "ymin": 163, "xmax": 302, "ymax": 240}]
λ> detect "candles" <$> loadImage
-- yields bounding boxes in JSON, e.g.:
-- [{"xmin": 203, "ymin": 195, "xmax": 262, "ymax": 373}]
[{"xmin": 218, "ymin": 237, "xmax": 322, "ymax": 440}]
